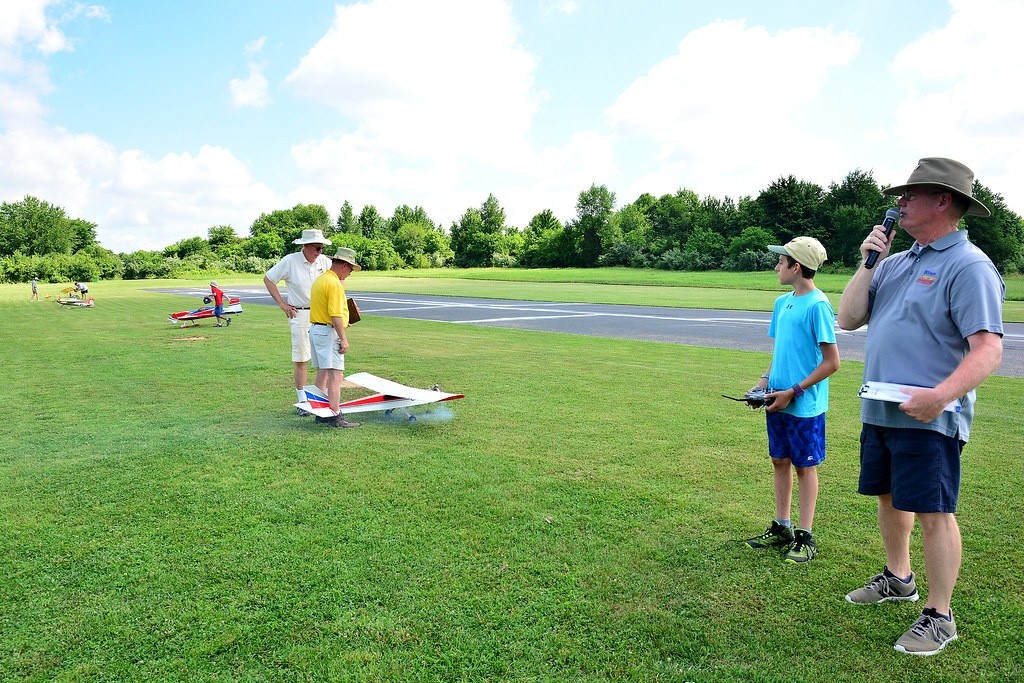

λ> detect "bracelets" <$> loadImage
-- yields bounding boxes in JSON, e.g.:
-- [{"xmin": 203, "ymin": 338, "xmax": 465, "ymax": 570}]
[{"xmin": 762, "ymin": 374, "xmax": 769, "ymax": 378}]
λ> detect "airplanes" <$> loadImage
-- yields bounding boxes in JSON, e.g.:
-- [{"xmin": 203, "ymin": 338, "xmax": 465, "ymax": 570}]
[
  {"xmin": 57, "ymin": 296, "xmax": 95, "ymax": 309},
  {"xmin": 167, "ymin": 298, "xmax": 243, "ymax": 329},
  {"xmin": 293, "ymin": 371, "xmax": 465, "ymax": 430}
]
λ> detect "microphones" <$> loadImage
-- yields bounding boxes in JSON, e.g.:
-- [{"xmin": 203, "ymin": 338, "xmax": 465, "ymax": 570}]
[{"xmin": 864, "ymin": 207, "xmax": 901, "ymax": 270}]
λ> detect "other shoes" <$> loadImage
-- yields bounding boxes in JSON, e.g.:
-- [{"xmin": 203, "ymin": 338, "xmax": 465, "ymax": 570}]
[
  {"xmin": 30, "ymin": 299, "xmax": 34, "ymax": 302},
  {"xmin": 38, "ymin": 299, "xmax": 42, "ymax": 301}
]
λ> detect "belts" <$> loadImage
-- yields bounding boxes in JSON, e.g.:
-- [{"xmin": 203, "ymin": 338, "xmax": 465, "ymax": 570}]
[
  {"xmin": 295, "ymin": 307, "xmax": 310, "ymax": 309},
  {"xmin": 314, "ymin": 322, "xmax": 334, "ymax": 328}
]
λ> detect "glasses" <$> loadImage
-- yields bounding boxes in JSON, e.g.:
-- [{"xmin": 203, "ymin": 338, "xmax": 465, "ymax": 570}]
[
  {"xmin": 901, "ymin": 189, "xmax": 921, "ymax": 202},
  {"xmin": 350, "ymin": 265, "xmax": 354, "ymax": 272},
  {"xmin": 313, "ymin": 246, "xmax": 324, "ymax": 250}
]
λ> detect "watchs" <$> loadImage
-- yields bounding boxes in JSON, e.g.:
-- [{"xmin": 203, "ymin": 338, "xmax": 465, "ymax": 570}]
[{"xmin": 793, "ymin": 384, "xmax": 804, "ymax": 397}]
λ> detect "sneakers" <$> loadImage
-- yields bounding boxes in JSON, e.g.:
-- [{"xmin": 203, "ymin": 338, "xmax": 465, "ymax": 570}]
[
  {"xmin": 315, "ymin": 416, "xmax": 329, "ymax": 424},
  {"xmin": 226, "ymin": 318, "xmax": 231, "ymax": 326},
  {"xmin": 845, "ymin": 565, "xmax": 919, "ymax": 606},
  {"xmin": 213, "ymin": 323, "xmax": 222, "ymax": 327},
  {"xmin": 786, "ymin": 528, "xmax": 817, "ymax": 564},
  {"xmin": 744, "ymin": 519, "xmax": 795, "ymax": 549},
  {"xmin": 329, "ymin": 411, "xmax": 360, "ymax": 428},
  {"xmin": 894, "ymin": 607, "xmax": 959, "ymax": 657},
  {"xmin": 296, "ymin": 406, "xmax": 310, "ymax": 416}
]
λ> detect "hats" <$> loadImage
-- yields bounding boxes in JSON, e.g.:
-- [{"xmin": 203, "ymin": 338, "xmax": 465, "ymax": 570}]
[
  {"xmin": 292, "ymin": 229, "xmax": 333, "ymax": 245},
  {"xmin": 210, "ymin": 281, "xmax": 218, "ymax": 287},
  {"xmin": 35, "ymin": 277, "xmax": 38, "ymax": 280},
  {"xmin": 768, "ymin": 236, "xmax": 827, "ymax": 272},
  {"xmin": 327, "ymin": 246, "xmax": 361, "ymax": 271},
  {"xmin": 883, "ymin": 157, "xmax": 991, "ymax": 217}
]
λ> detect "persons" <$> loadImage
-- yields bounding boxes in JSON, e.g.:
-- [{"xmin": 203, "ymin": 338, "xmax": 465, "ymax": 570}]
[
  {"xmin": 837, "ymin": 158, "xmax": 1005, "ymax": 653},
  {"xmin": 263, "ymin": 229, "xmax": 344, "ymax": 416},
  {"xmin": 744, "ymin": 237, "xmax": 841, "ymax": 563},
  {"xmin": 75, "ymin": 282, "xmax": 88, "ymax": 299},
  {"xmin": 31, "ymin": 277, "xmax": 41, "ymax": 301},
  {"xmin": 308, "ymin": 246, "xmax": 362, "ymax": 428},
  {"xmin": 208, "ymin": 281, "xmax": 231, "ymax": 327}
]
